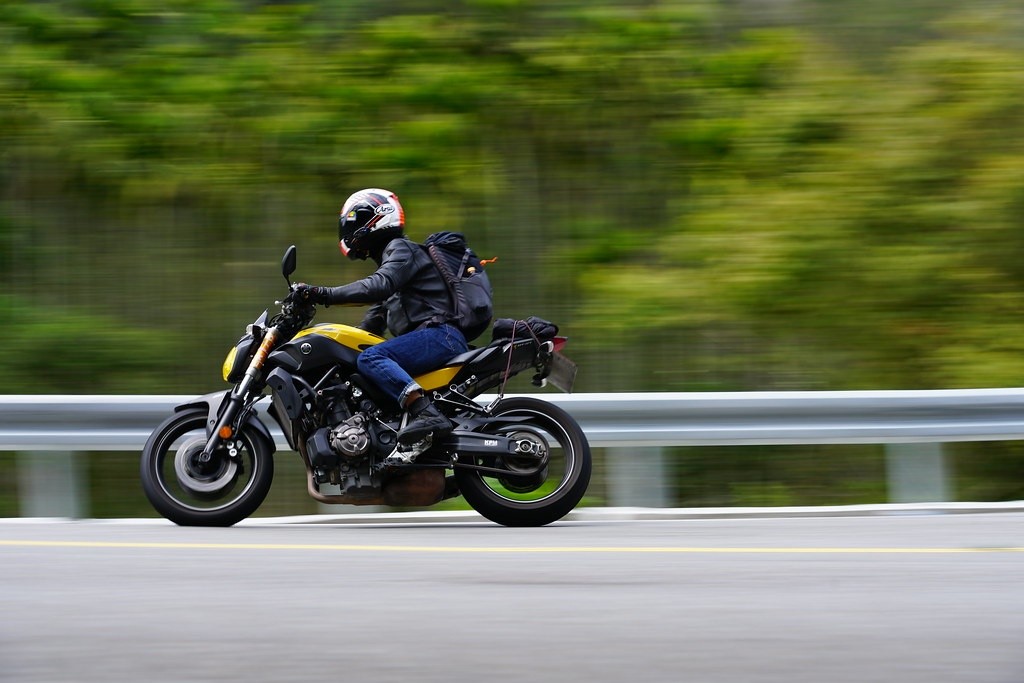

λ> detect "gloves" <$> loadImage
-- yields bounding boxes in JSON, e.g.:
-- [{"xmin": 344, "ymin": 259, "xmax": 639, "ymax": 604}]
[{"xmin": 287, "ymin": 282, "xmax": 318, "ymax": 308}]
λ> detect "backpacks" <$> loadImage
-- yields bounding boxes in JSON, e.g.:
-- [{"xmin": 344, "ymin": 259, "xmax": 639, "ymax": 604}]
[{"xmin": 407, "ymin": 231, "xmax": 498, "ymax": 343}]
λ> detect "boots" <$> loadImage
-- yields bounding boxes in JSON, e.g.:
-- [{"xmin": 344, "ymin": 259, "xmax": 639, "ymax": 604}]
[{"xmin": 396, "ymin": 395, "xmax": 453, "ymax": 443}]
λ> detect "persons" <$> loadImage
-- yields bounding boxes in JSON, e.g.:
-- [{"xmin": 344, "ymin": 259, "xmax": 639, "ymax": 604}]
[{"xmin": 281, "ymin": 188, "xmax": 467, "ymax": 440}]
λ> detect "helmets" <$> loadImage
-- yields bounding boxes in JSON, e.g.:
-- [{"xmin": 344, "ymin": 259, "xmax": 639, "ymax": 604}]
[{"xmin": 338, "ymin": 188, "xmax": 405, "ymax": 261}]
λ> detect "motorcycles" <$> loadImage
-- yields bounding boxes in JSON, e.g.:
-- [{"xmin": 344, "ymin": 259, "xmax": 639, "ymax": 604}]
[{"xmin": 140, "ymin": 245, "xmax": 592, "ymax": 527}]
